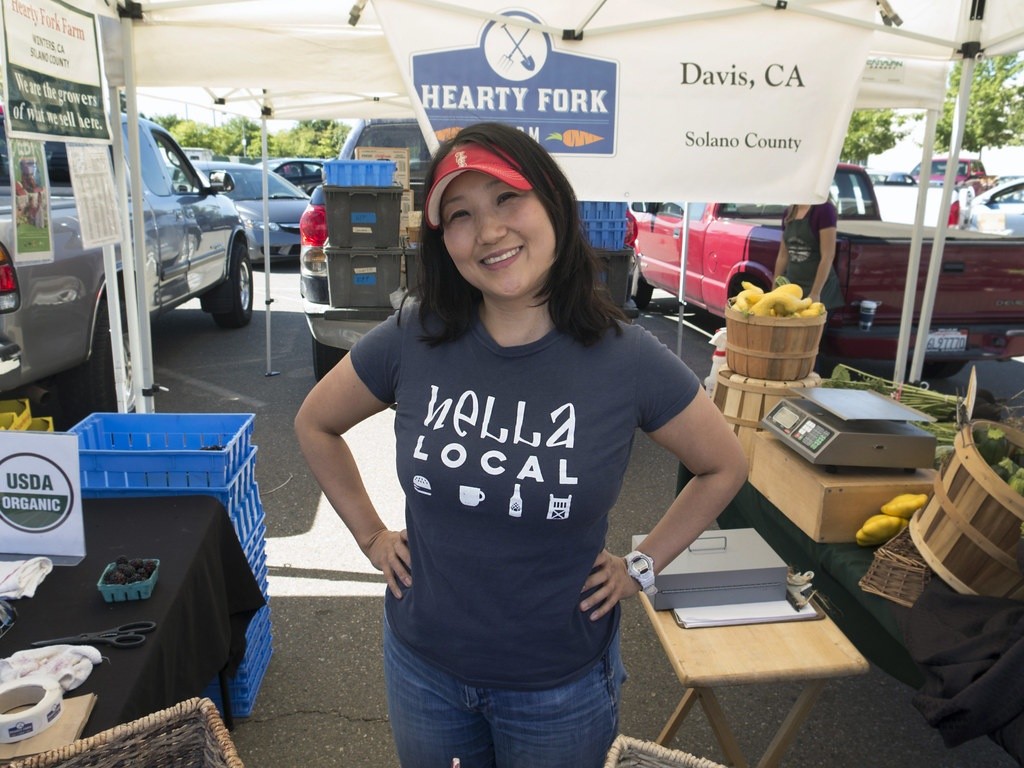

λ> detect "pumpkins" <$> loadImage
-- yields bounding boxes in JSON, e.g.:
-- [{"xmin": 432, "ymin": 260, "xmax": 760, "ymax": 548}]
[
  {"xmin": 856, "ymin": 494, "xmax": 927, "ymax": 545},
  {"xmin": 972, "ymin": 427, "xmax": 1024, "ymax": 498},
  {"xmin": 730, "ymin": 276, "xmax": 825, "ymax": 317}
]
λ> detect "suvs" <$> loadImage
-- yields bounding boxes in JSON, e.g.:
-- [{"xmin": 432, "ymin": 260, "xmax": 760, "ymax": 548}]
[{"xmin": 1, "ymin": 107, "xmax": 255, "ymax": 423}]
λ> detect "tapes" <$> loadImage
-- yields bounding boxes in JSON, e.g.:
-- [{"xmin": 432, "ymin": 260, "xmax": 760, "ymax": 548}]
[{"xmin": 1, "ymin": 676, "xmax": 66, "ymax": 743}]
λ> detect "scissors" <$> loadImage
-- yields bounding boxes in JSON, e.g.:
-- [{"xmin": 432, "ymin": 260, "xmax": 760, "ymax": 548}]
[{"xmin": 32, "ymin": 620, "xmax": 157, "ymax": 650}]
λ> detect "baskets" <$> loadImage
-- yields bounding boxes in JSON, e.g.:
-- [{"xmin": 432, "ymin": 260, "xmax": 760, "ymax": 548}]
[
  {"xmin": 858, "ymin": 525, "xmax": 932, "ymax": 607},
  {"xmin": 6, "ymin": 698, "xmax": 244, "ymax": 768},
  {"xmin": 604, "ymin": 734, "xmax": 727, "ymax": 768},
  {"xmin": 65, "ymin": 413, "xmax": 272, "ymax": 717}
]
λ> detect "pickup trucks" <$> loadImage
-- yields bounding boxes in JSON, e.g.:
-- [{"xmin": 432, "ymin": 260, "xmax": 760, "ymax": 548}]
[
  {"xmin": 628, "ymin": 162, "xmax": 1024, "ymax": 379},
  {"xmin": 296, "ymin": 116, "xmax": 632, "ymax": 384}
]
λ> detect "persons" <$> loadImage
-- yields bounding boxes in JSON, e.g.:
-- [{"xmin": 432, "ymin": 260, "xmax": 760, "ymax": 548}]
[
  {"xmin": 294, "ymin": 117, "xmax": 748, "ymax": 768},
  {"xmin": 772, "ymin": 191, "xmax": 846, "ymax": 359}
]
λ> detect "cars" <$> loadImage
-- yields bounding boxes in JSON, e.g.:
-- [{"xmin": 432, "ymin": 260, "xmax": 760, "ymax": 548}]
[
  {"xmin": 246, "ymin": 156, "xmax": 327, "ymax": 197},
  {"xmin": 863, "ymin": 157, "xmax": 1024, "ymax": 239},
  {"xmin": 167, "ymin": 159, "xmax": 311, "ymax": 265}
]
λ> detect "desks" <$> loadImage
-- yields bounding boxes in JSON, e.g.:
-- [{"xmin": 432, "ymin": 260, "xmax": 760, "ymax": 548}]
[
  {"xmin": 672, "ymin": 457, "xmax": 1024, "ymax": 767},
  {"xmin": 1, "ymin": 488, "xmax": 268, "ymax": 742},
  {"xmin": 638, "ymin": 591, "xmax": 870, "ymax": 768}
]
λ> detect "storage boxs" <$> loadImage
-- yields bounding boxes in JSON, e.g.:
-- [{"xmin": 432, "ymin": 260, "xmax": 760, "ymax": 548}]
[
  {"xmin": 59, "ymin": 409, "xmax": 273, "ymax": 718},
  {"xmin": 319, "ymin": 158, "xmax": 425, "ymax": 309}
]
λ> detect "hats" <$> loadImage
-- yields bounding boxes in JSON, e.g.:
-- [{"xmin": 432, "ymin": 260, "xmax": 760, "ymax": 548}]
[{"xmin": 425, "ymin": 140, "xmax": 548, "ymax": 225}]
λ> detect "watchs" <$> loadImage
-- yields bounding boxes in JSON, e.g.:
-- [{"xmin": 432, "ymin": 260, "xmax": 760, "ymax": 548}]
[{"xmin": 624, "ymin": 550, "xmax": 655, "ymax": 591}]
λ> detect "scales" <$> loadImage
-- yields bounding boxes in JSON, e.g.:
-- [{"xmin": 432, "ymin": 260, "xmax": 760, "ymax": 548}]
[{"xmin": 761, "ymin": 387, "xmax": 938, "ymax": 476}]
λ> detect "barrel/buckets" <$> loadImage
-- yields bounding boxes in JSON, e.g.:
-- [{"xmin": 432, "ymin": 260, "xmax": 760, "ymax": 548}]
[
  {"xmin": 908, "ymin": 418, "xmax": 1023, "ymax": 601},
  {"xmin": 725, "ymin": 297, "xmax": 827, "ymax": 381},
  {"xmin": 709, "ymin": 360, "xmax": 822, "ymax": 477}
]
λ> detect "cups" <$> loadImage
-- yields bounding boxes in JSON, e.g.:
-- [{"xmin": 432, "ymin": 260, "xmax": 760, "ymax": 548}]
[{"xmin": 859, "ymin": 300, "xmax": 877, "ymax": 331}]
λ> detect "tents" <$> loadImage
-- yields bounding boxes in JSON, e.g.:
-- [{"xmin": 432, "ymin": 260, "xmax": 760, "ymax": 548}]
[{"xmin": 0, "ymin": 0, "xmax": 1023, "ymax": 414}]
